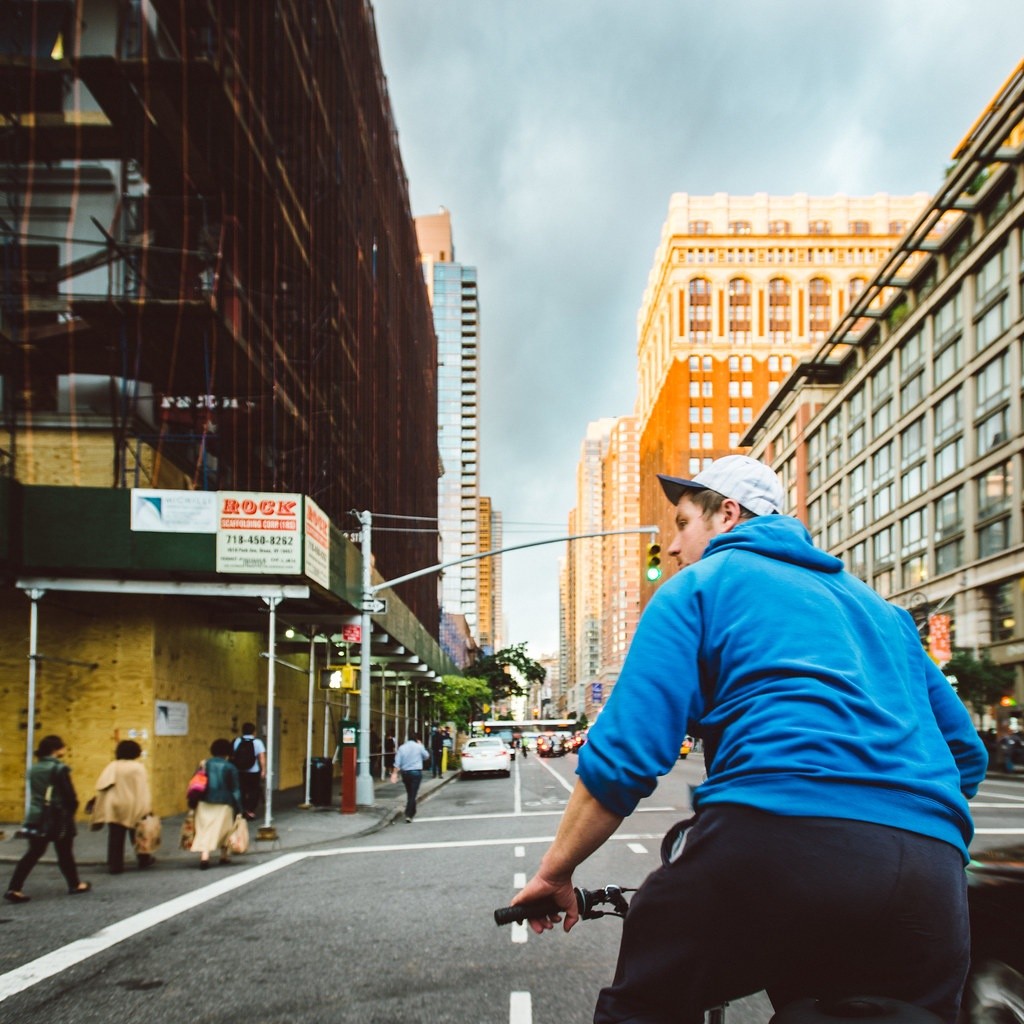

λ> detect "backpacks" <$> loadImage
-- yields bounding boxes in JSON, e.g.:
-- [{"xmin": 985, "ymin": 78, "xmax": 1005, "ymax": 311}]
[{"xmin": 234, "ymin": 736, "xmax": 256, "ymax": 769}]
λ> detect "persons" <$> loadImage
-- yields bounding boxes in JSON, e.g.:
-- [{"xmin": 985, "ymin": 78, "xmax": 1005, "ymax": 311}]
[
  {"xmin": 510, "ymin": 455, "xmax": 990, "ymax": 1024},
  {"xmin": 231, "ymin": 721, "xmax": 271, "ymax": 818},
  {"xmin": 189, "ymin": 738, "xmax": 254, "ymax": 870},
  {"xmin": 380, "ymin": 729, "xmax": 396, "ymax": 775},
  {"xmin": 0, "ymin": 735, "xmax": 93, "ymax": 902},
  {"xmin": 390, "ymin": 733, "xmax": 429, "ymax": 822},
  {"xmin": 520, "ymin": 735, "xmax": 528, "ymax": 759},
  {"xmin": 431, "ymin": 725, "xmax": 450, "ymax": 780},
  {"xmin": 370, "ymin": 724, "xmax": 382, "ymax": 775},
  {"xmin": 91, "ymin": 740, "xmax": 157, "ymax": 874}
]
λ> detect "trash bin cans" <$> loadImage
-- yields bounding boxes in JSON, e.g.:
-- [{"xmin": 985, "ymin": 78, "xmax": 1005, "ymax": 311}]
[{"xmin": 303, "ymin": 754, "xmax": 334, "ymax": 807}]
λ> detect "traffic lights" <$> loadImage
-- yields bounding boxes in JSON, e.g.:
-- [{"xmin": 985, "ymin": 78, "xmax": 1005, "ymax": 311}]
[{"xmin": 646, "ymin": 540, "xmax": 664, "ymax": 581}]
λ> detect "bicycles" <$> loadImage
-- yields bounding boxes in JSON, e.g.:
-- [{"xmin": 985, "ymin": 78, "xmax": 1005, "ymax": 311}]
[{"xmin": 494, "ymin": 884, "xmax": 730, "ymax": 1024}]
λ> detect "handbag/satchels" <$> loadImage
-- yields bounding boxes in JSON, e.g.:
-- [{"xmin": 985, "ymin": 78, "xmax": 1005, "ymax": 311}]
[
  {"xmin": 57, "ymin": 800, "xmax": 72, "ymax": 820},
  {"xmin": 186, "ymin": 767, "xmax": 207, "ymax": 798},
  {"xmin": 133, "ymin": 815, "xmax": 163, "ymax": 854},
  {"xmin": 180, "ymin": 817, "xmax": 195, "ymax": 850},
  {"xmin": 221, "ymin": 814, "xmax": 250, "ymax": 855}
]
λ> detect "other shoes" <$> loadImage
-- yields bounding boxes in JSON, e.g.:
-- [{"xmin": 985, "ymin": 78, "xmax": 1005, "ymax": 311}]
[
  {"xmin": 69, "ymin": 881, "xmax": 90, "ymax": 894},
  {"xmin": 138, "ymin": 854, "xmax": 152, "ymax": 869},
  {"xmin": 102, "ymin": 864, "xmax": 119, "ymax": 872},
  {"xmin": 245, "ymin": 812, "xmax": 257, "ymax": 821},
  {"xmin": 405, "ymin": 817, "xmax": 412, "ymax": 823},
  {"xmin": 4, "ymin": 890, "xmax": 31, "ymax": 902},
  {"xmin": 201, "ymin": 860, "xmax": 207, "ymax": 867}
]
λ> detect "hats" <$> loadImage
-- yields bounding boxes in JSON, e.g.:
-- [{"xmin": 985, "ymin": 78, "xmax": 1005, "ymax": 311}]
[{"xmin": 657, "ymin": 455, "xmax": 783, "ymax": 515}]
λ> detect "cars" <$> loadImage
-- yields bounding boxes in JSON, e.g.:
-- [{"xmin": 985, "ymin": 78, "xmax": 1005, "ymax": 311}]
[
  {"xmin": 460, "ymin": 737, "xmax": 515, "ymax": 778},
  {"xmin": 536, "ymin": 731, "xmax": 593, "ymax": 758}
]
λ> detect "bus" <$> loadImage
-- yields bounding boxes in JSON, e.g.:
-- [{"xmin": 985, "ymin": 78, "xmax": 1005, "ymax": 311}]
[{"xmin": 485, "ymin": 719, "xmax": 576, "ymax": 747}]
